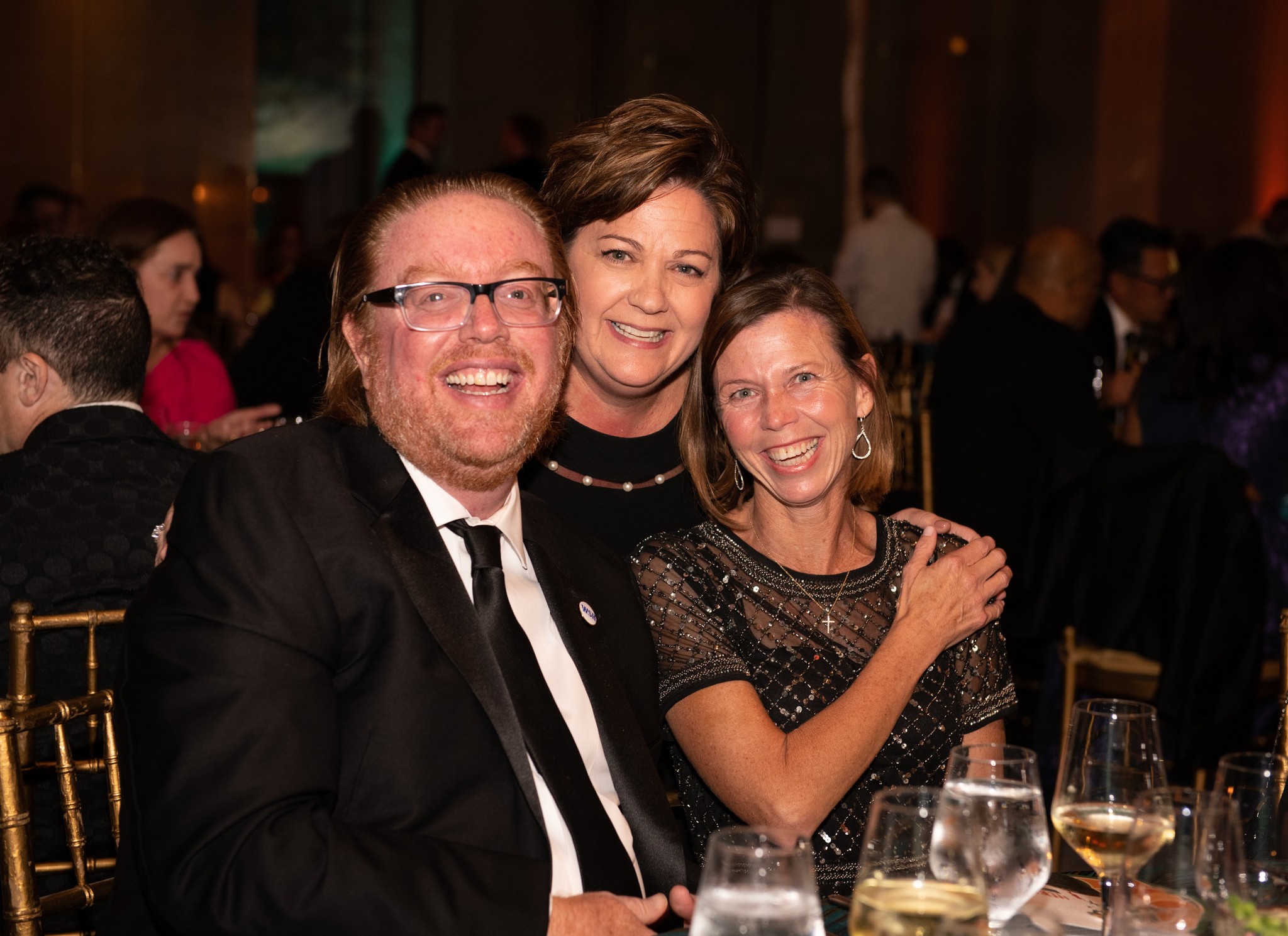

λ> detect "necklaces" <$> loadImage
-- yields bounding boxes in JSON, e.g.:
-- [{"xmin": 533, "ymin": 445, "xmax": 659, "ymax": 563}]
[{"xmin": 748, "ymin": 494, "xmax": 856, "ymax": 635}]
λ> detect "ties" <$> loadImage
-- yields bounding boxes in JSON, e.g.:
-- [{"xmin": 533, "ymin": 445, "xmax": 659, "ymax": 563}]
[
  {"xmin": 1113, "ymin": 330, "xmax": 1141, "ymax": 439},
  {"xmin": 447, "ymin": 516, "xmax": 644, "ymax": 901}
]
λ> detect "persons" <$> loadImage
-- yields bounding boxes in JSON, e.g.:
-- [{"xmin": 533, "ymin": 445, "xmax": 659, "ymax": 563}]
[
  {"xmin": 826, "ymin": 165, "xmax": 940, "ymax": 386},
  {"xmin": 517, "ymin": 92, "xmax": 985, "ymax": 935},
  {"xmin": 629, "ymin": 261, "xmax": 1020, "ymax": 899},
  {"xmin": 381, "ymin": 99, "xmax": 448, "ymax": 188},
  {"xmin": 1, "ymin": 234, "xmax": 210, "ymax": 934},
  {"xmin": 0, "ymin": 181, "xmax": 70, "ymax": 238},
  {"xmin": 98, "ymin": 170, "xmax": 698, "ymax": 935},
  {"xmin": 923, "ymin": 197, "xmax": 1288, "ymax": 876},
  {"xmin": 491, "ymin": 111, "xmax": 551, "ymax": 193},
  {"xmin": 98, "ymin": 198, "xmax": 288, "ymax": 454}
]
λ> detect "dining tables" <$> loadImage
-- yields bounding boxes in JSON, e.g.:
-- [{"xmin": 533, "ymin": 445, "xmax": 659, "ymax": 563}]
[{"xmin": 651, "ymin": 861, "xmax": 1287, "ymax": 936}]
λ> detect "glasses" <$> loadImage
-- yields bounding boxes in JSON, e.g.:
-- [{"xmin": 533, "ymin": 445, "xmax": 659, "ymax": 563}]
[
  {"xmin": 355, "ymin": 277, "xmax": 570, "ymax": 332},
  {"xmin": 1126, "ymin": 270, "xmax": 1180, "ymax": 291}
]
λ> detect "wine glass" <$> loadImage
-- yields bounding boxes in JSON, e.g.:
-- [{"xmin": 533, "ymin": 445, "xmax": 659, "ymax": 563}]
[{"xmin": 682, "ymin": 686, "xmax": 1287, "ymax": 934}]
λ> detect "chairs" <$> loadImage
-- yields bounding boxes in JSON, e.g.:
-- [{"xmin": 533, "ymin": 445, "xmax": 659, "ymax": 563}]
[
  {"xmin": 0, "ymin": 690, "xmax": 127, "ymax": 936},
  {"xmin": 3, "ymin": 597, "xmax": 127, "ymax": 880}
]
